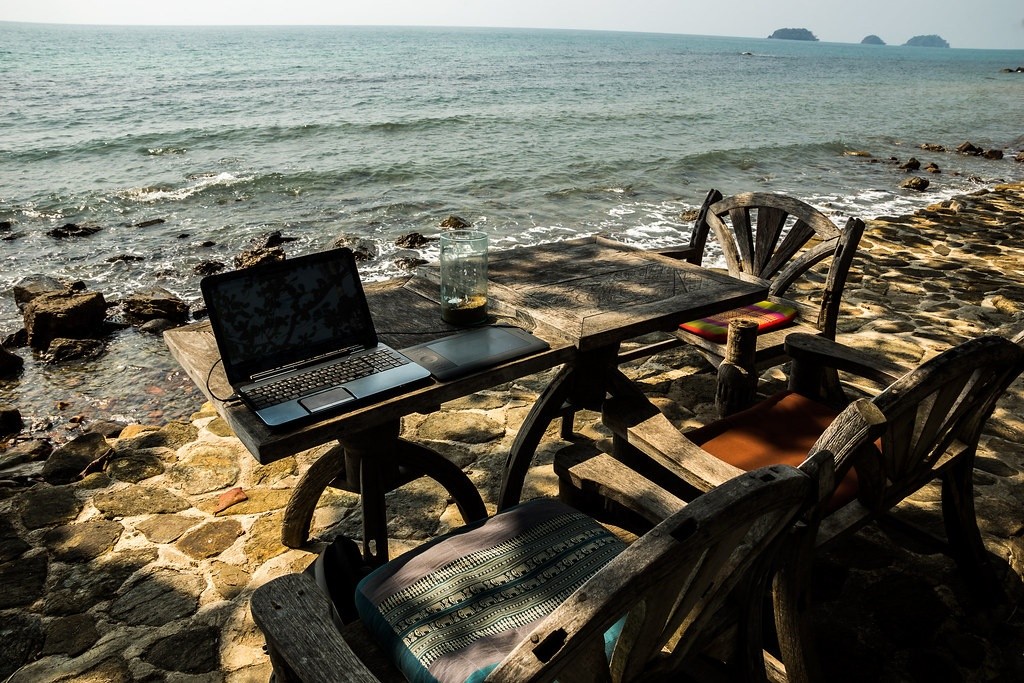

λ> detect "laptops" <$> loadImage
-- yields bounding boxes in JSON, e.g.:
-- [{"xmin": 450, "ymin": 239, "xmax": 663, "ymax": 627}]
[{"xmin": 201, "ymin": 247, "xmax": 431, "ymax": 430}]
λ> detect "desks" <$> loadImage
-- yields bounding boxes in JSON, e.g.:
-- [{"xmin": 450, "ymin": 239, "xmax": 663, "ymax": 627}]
[{"xmin": 162, "ymin": 234, "xmax": 770, "ymax": 571}]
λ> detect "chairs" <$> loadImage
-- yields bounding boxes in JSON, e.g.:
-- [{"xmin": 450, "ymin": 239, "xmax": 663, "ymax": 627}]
[
  {"xmin": 602, "ymin": 333, "xmax": 1024, "ymax": 683},
  {"xmin": 252, "ymin": 440, "xmax": 811, "ymax": 683},
  {"xmin": 616, "ymin": 188, "xmax": 864, "ymax": 407}
]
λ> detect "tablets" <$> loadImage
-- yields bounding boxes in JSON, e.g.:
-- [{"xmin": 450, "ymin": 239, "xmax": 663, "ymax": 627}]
[{"xmin": 398, "ymin": 322, "xmax": 550, "ymax": 383}]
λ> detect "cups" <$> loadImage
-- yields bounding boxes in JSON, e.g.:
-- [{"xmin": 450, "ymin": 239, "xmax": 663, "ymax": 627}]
[{"xmin": 441, "ymin": 230, "xmax": 488, "ymax": 325}]
